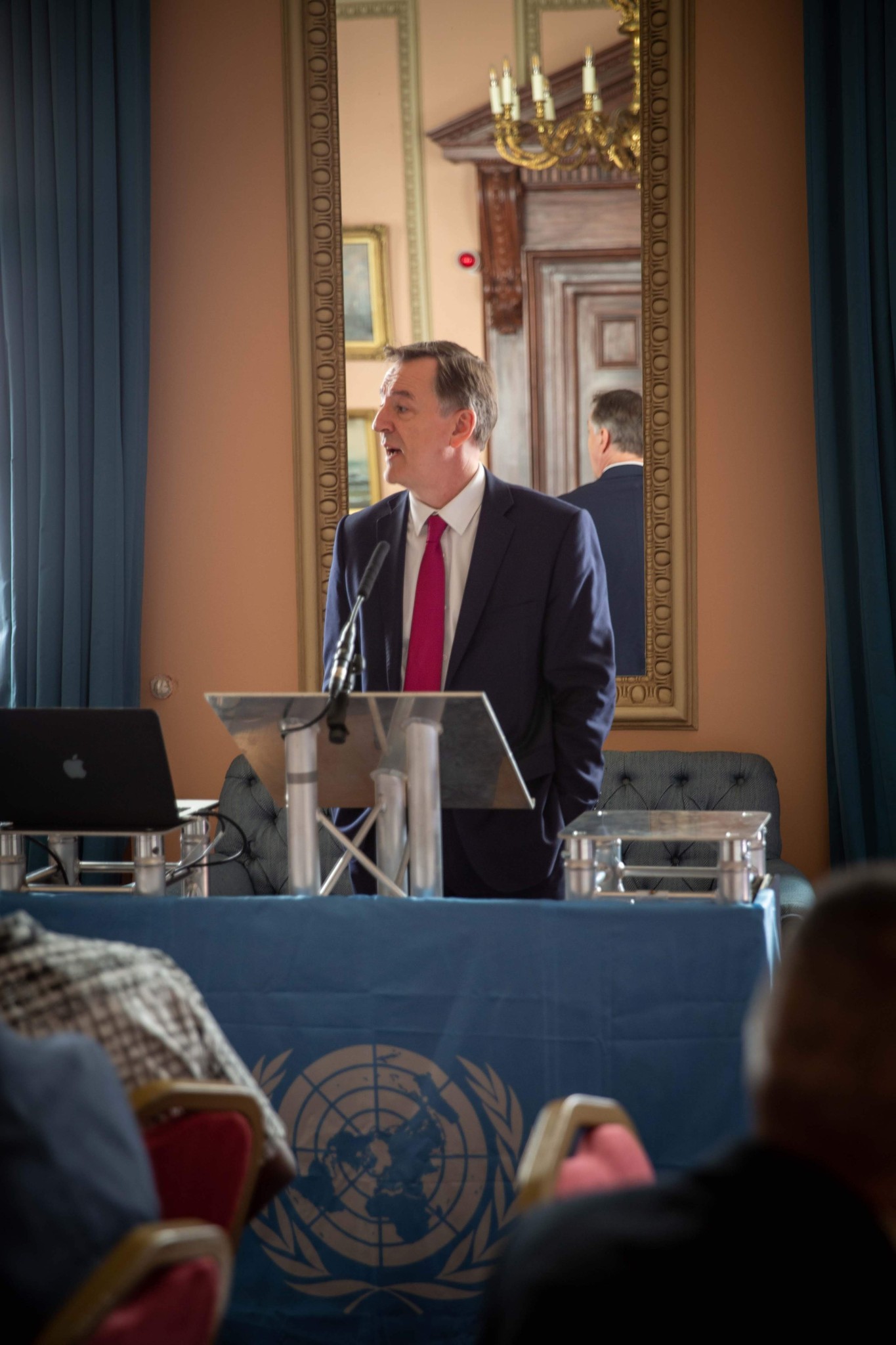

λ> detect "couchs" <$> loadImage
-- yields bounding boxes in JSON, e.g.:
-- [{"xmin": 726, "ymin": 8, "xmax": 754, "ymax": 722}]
[{"xmin": 205, "ymin": 747, "xmax": 782, "ymax": 901}]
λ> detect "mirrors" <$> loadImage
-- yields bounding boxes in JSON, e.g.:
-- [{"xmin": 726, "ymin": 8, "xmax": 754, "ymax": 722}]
[{"xmin": 275, "ymin": 2, "xmax": 697, "ymax": 730}]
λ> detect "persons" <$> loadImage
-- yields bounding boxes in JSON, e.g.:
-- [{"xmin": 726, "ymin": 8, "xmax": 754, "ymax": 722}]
[
  {"xmin": 487, "ymin": 868, "xmax": 896, "ymax": 1345},
  {"xmin": 316, "ymin": 340, "xmax": 617, "ymax": 898},
  {"xmin": 1, "ymin": 1029, "xmax": 162, "ymax": 1344},
  {"xmin": 558, "ymin": 389, "xmax": 647, "ymax": 678},
  {"xmin": 0, "ymin": 912, "xmax": 298, "ymax": 1235}
]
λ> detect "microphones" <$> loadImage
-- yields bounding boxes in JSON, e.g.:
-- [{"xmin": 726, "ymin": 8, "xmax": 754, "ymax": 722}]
[{"xmin": 327, "ymin": 540, "xmax": 390, "ymax": 701}]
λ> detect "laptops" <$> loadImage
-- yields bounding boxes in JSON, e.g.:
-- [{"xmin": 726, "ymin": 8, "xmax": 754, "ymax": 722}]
[{"xmin": 0, "ymin": 706, "xmax": 221, "ymax": 832}]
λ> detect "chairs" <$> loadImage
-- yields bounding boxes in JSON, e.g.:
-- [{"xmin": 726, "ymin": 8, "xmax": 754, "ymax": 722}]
[
  {"xmin": 130, "ymin": 1077, "xmax": 268, "ymax": 1243},
  {"xmin": 36, "ymin": 1218, "xmax": 235, "ymax": 1344}
]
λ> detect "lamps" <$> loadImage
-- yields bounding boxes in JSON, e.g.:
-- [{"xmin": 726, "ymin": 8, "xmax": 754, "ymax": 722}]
[{"xmin": 487, "ymin": 1, "xmax": 645, "ymax": 188}]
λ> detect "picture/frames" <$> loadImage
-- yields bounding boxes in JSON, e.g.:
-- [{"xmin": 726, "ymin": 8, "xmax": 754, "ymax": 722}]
[
  {"xmin": 342, "ymin": 223, "xmax": 394, "ymax": 363},
  {"xmin": 346, "ymin": 407, "xmax": 382, "ymax": 514}
]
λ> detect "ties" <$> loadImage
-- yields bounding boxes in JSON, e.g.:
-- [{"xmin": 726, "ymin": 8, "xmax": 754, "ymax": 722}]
[{"xmin": 402, "ymin": 513, "xmax": 449, "ymax": 693}]
